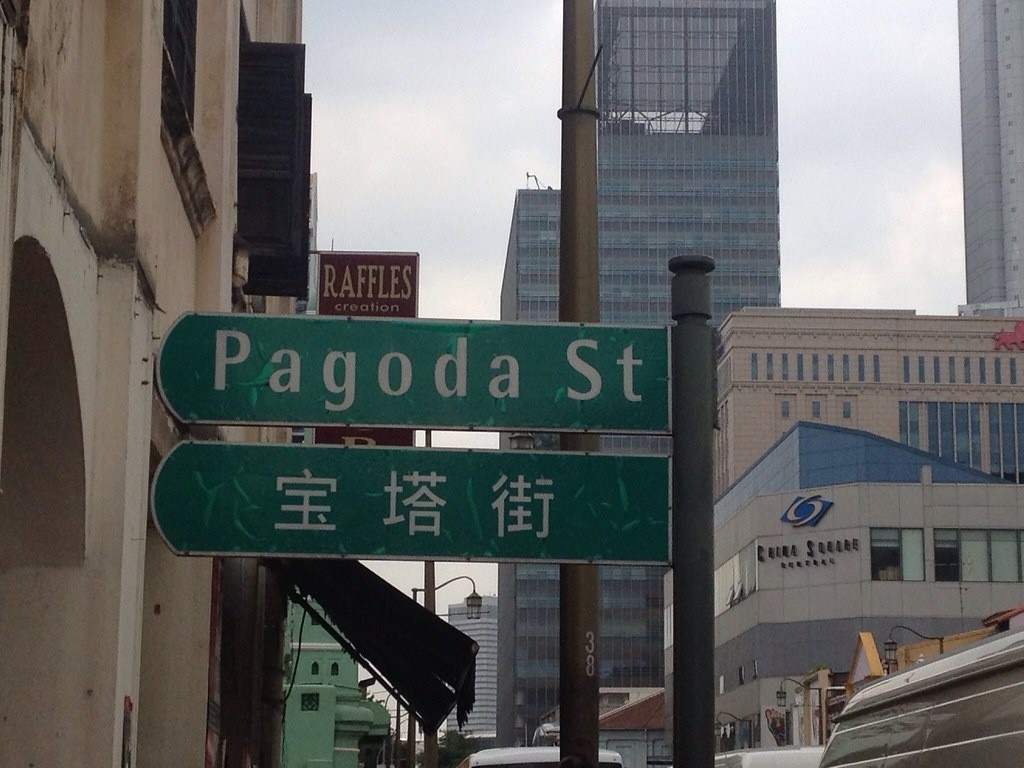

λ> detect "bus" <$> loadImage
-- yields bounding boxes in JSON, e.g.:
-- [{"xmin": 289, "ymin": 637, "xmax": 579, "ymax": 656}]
[{"xmin": 819, "ymin": 628, "xmax": 1024, "ymax": 768}]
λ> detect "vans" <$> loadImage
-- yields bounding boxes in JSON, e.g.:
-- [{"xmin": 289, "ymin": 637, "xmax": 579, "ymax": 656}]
[
  {"xmin": 714, "ymin": 747, "xmax": 824, "ymax": 767},
  {"xmin": 456, "ymin": 746, "xmax": 625, "ymax": 768}
]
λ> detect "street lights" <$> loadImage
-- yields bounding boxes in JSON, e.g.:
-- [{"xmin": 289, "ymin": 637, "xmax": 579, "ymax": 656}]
[
  {"xmin": 407, "ymin": 576, "xmax": 483, "ymax": 768},
  {"xmin": 716, "ymin": 712, "xmax": 755, "ymax": 751},
  {"xmin": 883, "ymin": 625, "xmax": 947, "ymax": 660},
  {"xmin": 775, "ymin": 678, "xmax": 825, "ymax": 746}
]
